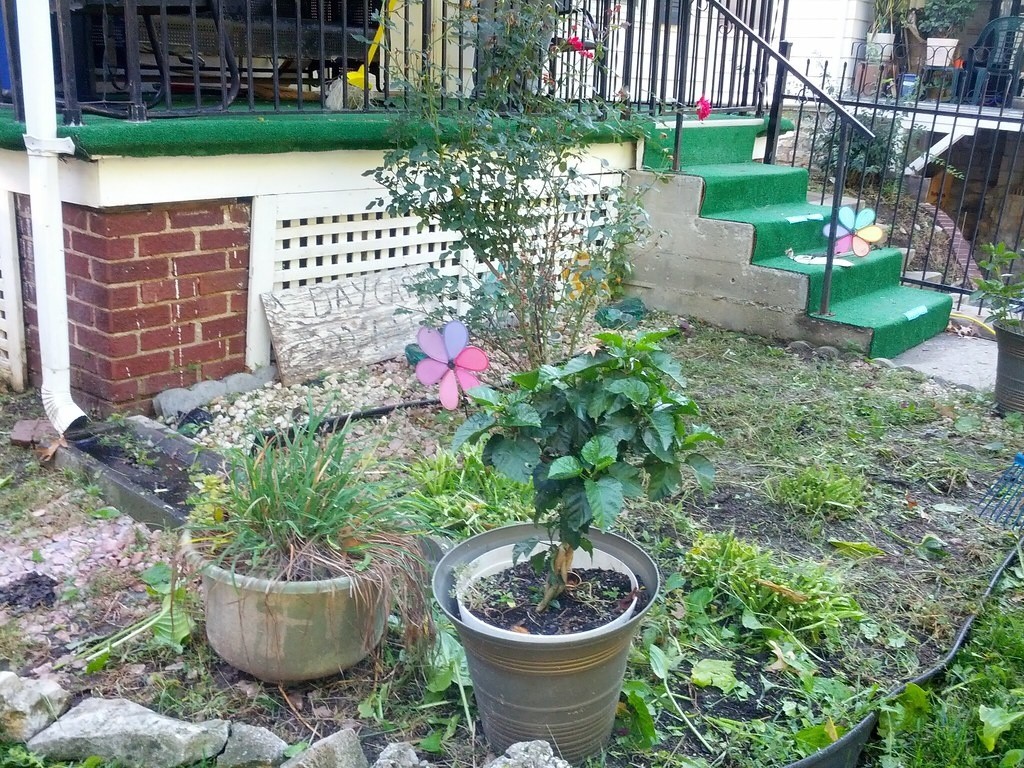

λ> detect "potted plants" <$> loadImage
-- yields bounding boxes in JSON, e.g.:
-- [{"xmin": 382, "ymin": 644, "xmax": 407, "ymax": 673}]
[
  {"xmin": 854, "ymin": 0, "xmax": 909, "ymax": 60},
  {"xmin": 175, "ymin": 387, "xmax": 437, "ymax": 685},
  {"xmin": 450, "ymin": 325, "xmax": 731, "ymax": 643},
  {"xmin": 918, "ymin": 0, "xmax": 977, "ymax": 67},
  {"xmin": 927, "ymin": 72, "xmax": 949, "ymax": 102},
  {"xmin": 969, "ymin": 241, "xmax": 1024, "ymax": 415}
]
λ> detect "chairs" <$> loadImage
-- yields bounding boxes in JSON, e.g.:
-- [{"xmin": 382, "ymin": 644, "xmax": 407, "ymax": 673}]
[
  {"xmin": 965, "ymin": 16, "xmax": 1024, "ymax": 107},
  {"xmin": 541, "ymin": 4, "xmax": 608, "ymax": 106}
]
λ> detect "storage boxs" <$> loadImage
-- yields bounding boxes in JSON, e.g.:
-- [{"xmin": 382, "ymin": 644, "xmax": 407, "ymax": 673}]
[{"xmin": 898, "ymin": 73, "xmax": 917, "ymax": 98}]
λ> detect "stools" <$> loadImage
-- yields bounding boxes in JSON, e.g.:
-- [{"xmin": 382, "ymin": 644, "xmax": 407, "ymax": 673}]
[{"xmin": 918, "ymin": 66, "xmax": 959, "ymax": 103}]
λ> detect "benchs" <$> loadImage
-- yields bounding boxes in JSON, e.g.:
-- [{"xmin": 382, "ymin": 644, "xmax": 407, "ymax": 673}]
[{"xmin": 85, "ymin": 0, "xmax": 390, "ymax": 117}]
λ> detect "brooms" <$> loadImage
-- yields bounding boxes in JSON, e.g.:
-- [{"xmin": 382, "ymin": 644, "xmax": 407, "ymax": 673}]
[{"xmin": 324, "ymin": 0, "xmax": 400, "ymax": 112}]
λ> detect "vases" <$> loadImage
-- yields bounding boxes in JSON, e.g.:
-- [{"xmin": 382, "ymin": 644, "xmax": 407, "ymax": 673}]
[{"xmin": 432, "ymin": 522, "xmax": 661, "ymax": 765}]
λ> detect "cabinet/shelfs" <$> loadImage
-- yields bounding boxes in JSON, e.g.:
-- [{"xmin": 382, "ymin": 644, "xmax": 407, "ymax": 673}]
[{"xmin": 855, "ymin": 61, "xmax": 895, "ymax": 99}]
